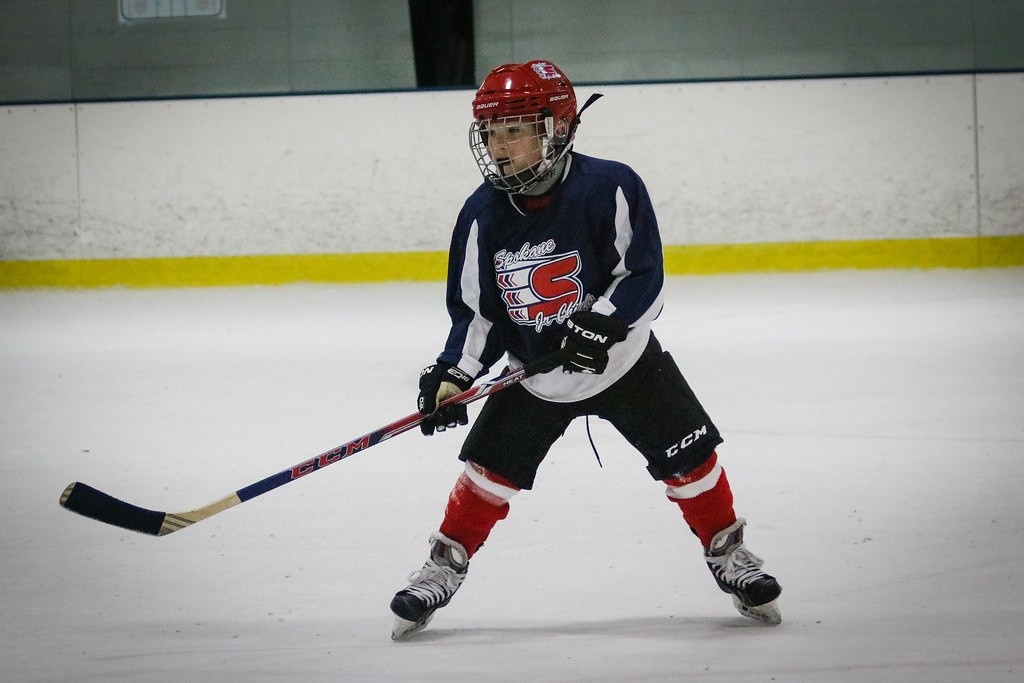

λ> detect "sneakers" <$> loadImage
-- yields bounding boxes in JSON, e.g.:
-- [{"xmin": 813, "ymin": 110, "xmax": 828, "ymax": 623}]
[
  {"xmin": 704, "ymin": 517, "xmax": 782, "ymax": 625},
  {"xmin": 390, "ymin": 531, "xmax": 469, "ymax": 640}
]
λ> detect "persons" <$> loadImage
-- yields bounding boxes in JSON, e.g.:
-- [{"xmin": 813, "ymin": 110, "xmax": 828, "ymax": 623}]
[{"xmin": 389, "ymin": 59, "xmax": 781, "ymax": 639}]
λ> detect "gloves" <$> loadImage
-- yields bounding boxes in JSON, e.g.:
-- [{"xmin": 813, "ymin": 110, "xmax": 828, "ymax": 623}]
[
  {"xmin": 548, "ymin": 309, "xmax": 629, "ymax": 374},
  {"xmin": 417, "ymin": 364, "xmax": 474, "ymax": 436}
]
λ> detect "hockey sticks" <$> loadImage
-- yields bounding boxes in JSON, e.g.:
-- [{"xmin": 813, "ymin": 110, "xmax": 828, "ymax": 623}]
[{"xmin": 56, "ymin": 347, "xmax": 567, "ymax": 539}]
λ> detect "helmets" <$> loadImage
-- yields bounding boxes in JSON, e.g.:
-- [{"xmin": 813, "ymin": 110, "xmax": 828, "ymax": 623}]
[{"xmin": 468, "ymin": 59, "xmax": 577, "ymax": 196}]
